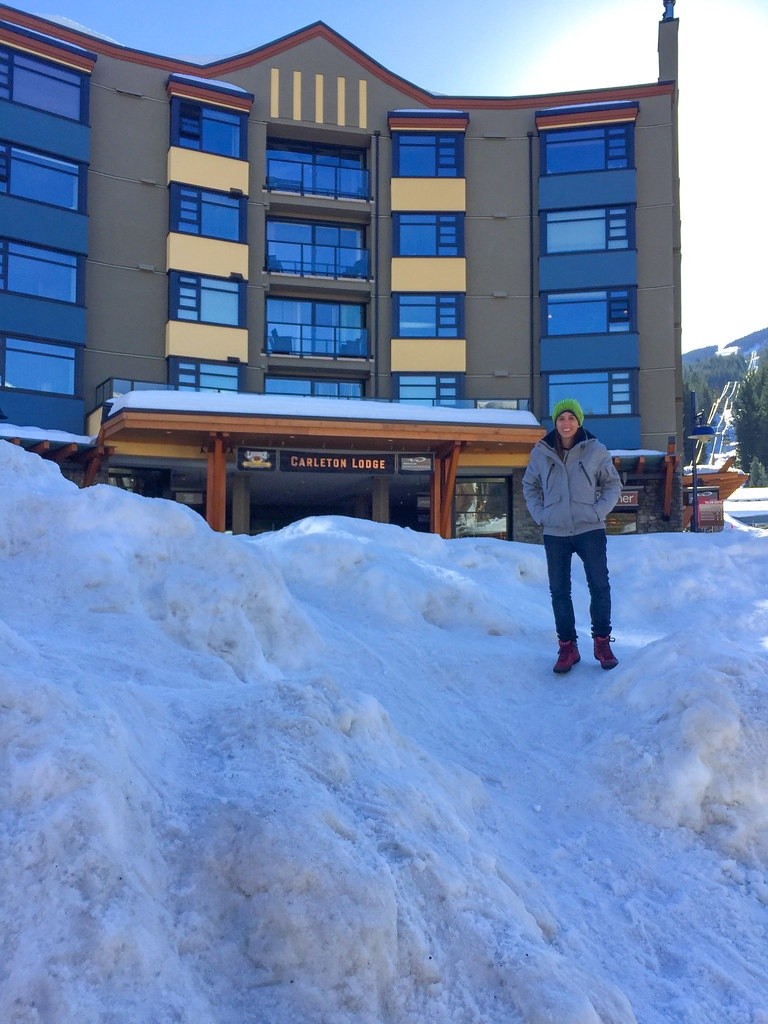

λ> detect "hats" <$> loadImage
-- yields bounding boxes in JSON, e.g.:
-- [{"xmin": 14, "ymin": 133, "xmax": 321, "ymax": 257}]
[{"xmin": 552, "ymin": 398, "xmax": 584, "ymax": 427}]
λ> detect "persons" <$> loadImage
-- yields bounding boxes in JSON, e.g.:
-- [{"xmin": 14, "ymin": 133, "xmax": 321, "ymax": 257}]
[{"xmin": 522, "ymin": 398, "xmax": 622, "ymax": 673}]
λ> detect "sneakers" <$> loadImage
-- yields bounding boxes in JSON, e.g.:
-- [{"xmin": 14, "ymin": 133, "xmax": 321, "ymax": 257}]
[
  {"xmin": 594, "ymin": 636, "xmax": 619, "ymax": 670},
  {"xmin": 553, "ymin": 641, "xmax": 581, "ymax": 674}
]
